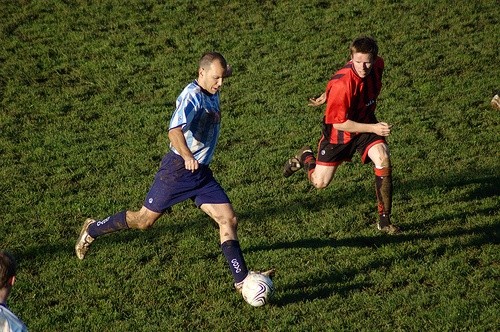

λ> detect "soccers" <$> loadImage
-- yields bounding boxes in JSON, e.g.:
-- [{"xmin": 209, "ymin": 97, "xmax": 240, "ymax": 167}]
[{"xmin": 242, "ymin": 273, "xmax": 274, "ymax": 306}]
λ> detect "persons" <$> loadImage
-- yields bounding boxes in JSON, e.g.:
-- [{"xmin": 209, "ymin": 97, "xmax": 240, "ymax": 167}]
[
  {"xmin": 75, "ymin": 53, "xmax": 276, "ymax": 294},
  {"xmin": 0, "ymin": 250, "xmax": 30, "ymax": 332},
  {"xmin": 282, "ymin": 37, "xmax": 401, "ymax": 234}
]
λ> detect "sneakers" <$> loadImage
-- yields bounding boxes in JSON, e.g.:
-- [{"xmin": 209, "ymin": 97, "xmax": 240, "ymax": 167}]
[
  {"xmin": 74, "ymin": 217, "xmax": 96, "ymax": 260},
  {"xmin": 282, "ymin": 146, "xmax": 314, "ymax": 178},
  {"xmin": 234, "ymin": 269, "xmax": 276, "ymax": 295},
  {"xmin": 378, "ymin": 222, "xmax": 401, "ymax": 236}
]
